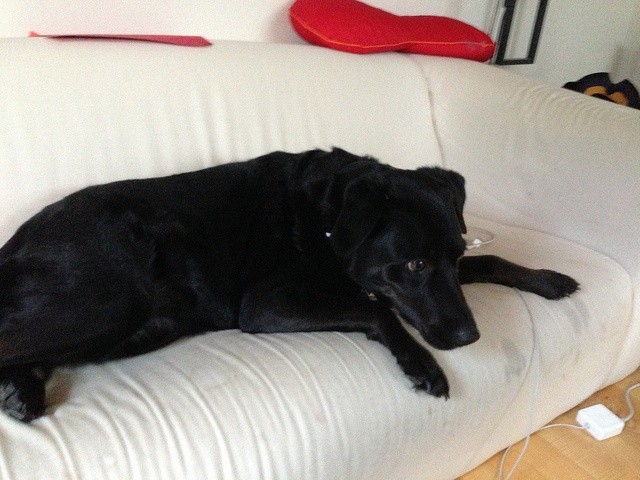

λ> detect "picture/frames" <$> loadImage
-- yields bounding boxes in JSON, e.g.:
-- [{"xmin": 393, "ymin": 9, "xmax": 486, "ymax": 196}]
[{"xmin": 494, "ymin": 1, "xmax": 549, "ymax": 66}]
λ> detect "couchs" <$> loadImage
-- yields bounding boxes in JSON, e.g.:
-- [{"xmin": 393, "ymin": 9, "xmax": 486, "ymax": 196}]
[{"xmin": 3, "ymin": 41, "xmax": 640, "ymax": 479}]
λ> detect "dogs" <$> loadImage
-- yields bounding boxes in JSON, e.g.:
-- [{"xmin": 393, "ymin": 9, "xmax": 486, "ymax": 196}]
[{"xmin": 0, "ymin": 146, "xmax": 581, "ymax": 425}]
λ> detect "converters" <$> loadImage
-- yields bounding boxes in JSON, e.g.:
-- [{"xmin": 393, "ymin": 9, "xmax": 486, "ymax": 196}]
[{"xmin": 575, "ymin": 403, "xmax": 624, "ymax": 440}]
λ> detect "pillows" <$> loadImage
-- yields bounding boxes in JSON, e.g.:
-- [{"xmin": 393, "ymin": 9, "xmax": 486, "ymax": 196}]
[{"xmin": 289, "ymin": 1, "xmax": 498, "ymax": 63}]
